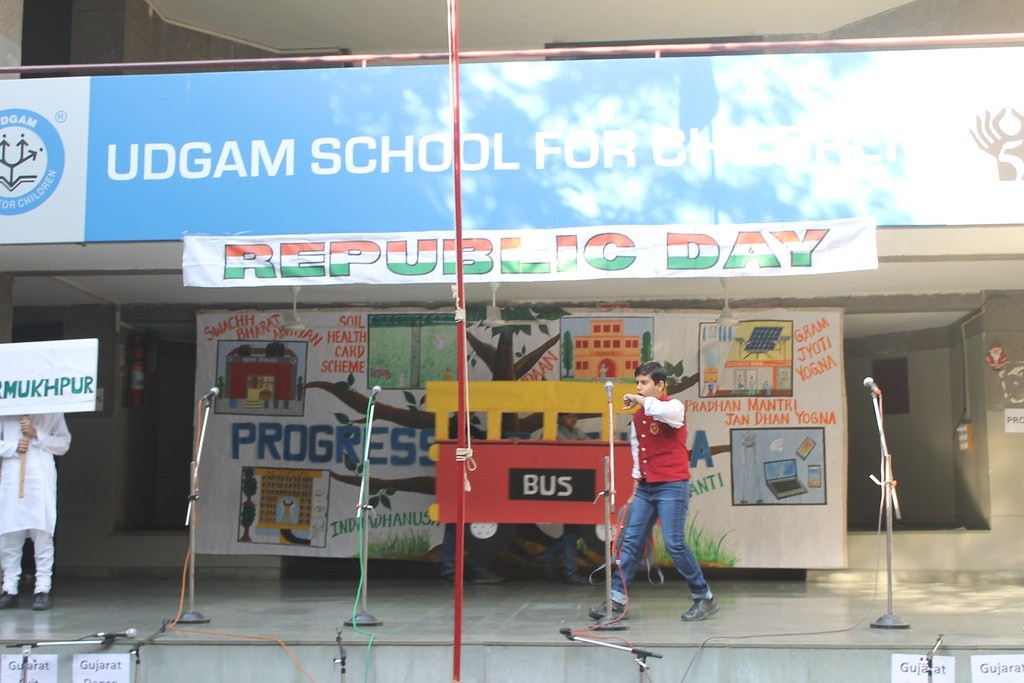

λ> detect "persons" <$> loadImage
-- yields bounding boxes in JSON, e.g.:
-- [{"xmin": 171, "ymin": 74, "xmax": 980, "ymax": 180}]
[
  {"xmin": 440, "ymin": 411, "xmax": 504, "ymax": 585},
  {"xmin": 0, "ymin": 413, "xmax": 72, "ymax": 611},
  {"xmin": 588, "ymin": 362, "xmax": 720, "ymax": 623},
  {"xmin": 539, "ymin": 413, "xmax": 593, "ymax": 585}
]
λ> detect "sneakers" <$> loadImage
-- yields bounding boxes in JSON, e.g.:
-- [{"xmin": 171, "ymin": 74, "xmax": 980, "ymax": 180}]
[
  {"xmin": 32, "ymin": 594, "xmax": 51, "ymax": 610},
  {"xmin": 680, "ymin": 596, "xmax": 720, "ymax": 621},
  {"xmin": 589, "ymin": 600, "xmax": 629, "ymax": 619},
  {"xmin": 0, "ymin": 594, "xmax": 19, "ymax": 608}
]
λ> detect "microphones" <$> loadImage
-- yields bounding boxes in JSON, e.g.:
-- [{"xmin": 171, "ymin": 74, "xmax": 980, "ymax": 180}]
[
  {"xmin": 370, "ymin": 385, "xmax": 381, "ymax": 399},
  {"xmin": 97, "ymin": 628, "xmax": 137, "ymax": 639},
  {"xmin": 863, "ymin": 377, "xmax": 881, "ymax": 396},
  {"xmin": 201, "ymin": 387, "xmax": 220, "ymax": 402},
  {"xmin": 605, "ymin": 382, "xmax": 614, "ymax": 400}
]
